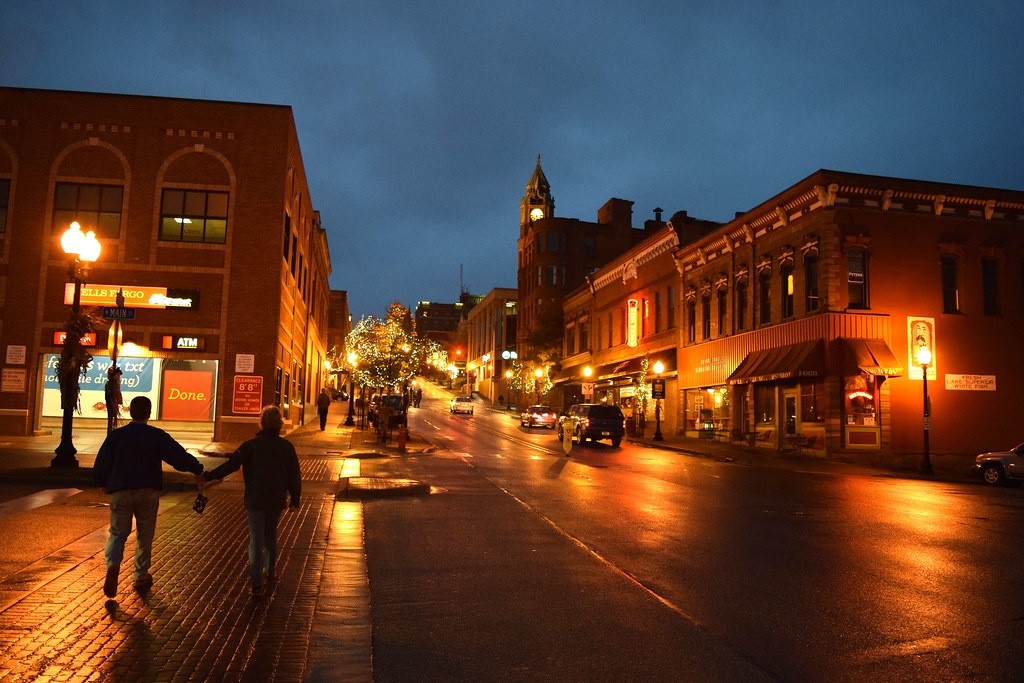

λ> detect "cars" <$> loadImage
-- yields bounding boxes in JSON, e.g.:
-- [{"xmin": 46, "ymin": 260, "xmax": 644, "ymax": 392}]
[
  {"xmin": 975, "ymin": 442, "xmax": 1024, "ymax": 486},
  {"xmin": 520, "ymin": 405, "xmax": 556, "ymax": 429},
  {"xmin": 369, "ymin": 394, "xmax": 407, "ymax": 429},
  {"xmin": 450, "ymin": 397, "xmax": 474, "ymax": 414}
]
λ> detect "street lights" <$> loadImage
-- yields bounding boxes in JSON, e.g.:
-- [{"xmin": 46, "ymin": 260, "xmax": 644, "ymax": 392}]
[
  {"xmin": 505, "ymin": 371, "xmax": 512, "ymax": 410},
  {"xmin": 653, "ymin": 360, "xmax": 665, "ymax": 441},
  {"xmin": 535, "ymin": 368, "xmax": 543, "ymax": 404},
  {"xmin": 49, "ymin": 220, "xmax": 103, "ymax": 468},
  {"xmin": 917, "ymin": 346, "xmax": 935, "ymax": 475}
]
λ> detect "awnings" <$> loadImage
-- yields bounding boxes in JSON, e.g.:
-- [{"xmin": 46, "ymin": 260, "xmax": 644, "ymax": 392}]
[
  {"xmin": 725, "ymin": 338, "xmax": 823, "ymax": 385},
  {"xmin": 830, "ymin": 337, "xmax": 903, "ymax": 378}
]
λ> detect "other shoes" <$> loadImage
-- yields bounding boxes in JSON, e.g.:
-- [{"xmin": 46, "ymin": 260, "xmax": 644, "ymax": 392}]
[
  {"xmin": 103, "ymin": 565, "xmax": 119, "ymax": 598},
  {"xmin": 135, "ymin": 572, "xmax": 153, "ymax": 589},
  {"xmin": 251, "ymin": 575, "xmax": 265, "ymax": 596},
  {"xmin": 265, "ymin": 576, "xmax": 276, "ymax": 598}
]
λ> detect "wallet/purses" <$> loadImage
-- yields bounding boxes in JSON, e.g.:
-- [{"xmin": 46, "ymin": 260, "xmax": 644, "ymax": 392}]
[{"xmin": 193, "ymin": 493, "xmax": 209, "ymax": 514}]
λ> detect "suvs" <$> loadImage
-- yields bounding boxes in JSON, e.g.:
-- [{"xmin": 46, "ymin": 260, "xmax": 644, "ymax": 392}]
[{"xmin": 558, "ymin": 403, "xmax": 626, "ymax": 448}]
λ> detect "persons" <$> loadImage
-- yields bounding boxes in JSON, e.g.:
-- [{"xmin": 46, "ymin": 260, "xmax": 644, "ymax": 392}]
[
  {"xmin": 410, "ymin": 388, "xmax": 422, "ymax": 408},
  {"xmin": 91, "ymin": 395, "xmax": 205, "ymax": 602},
  {"xmin": 316, "ymin": 389, "xmax": 330, "ymax": 430},
  {"xmin": 196, "ymin": 406, "xmax": 301, "ymax": 586},
  {"xmin": 498, "ymin": 393, "xmax": 504, "ymax": 406}
]
